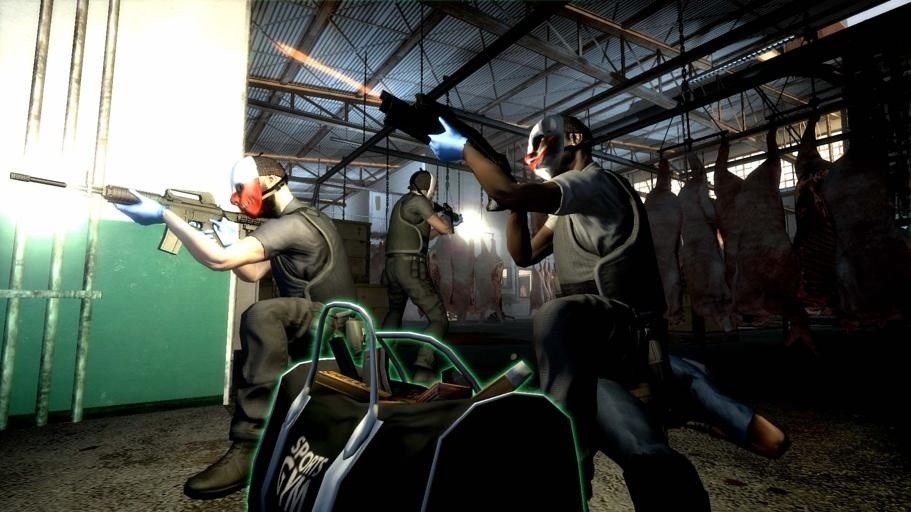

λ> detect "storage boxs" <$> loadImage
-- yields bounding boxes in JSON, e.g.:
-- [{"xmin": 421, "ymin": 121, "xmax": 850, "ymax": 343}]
[
  {"xmin": 355, "ymin": 284, "xmax": 392, "ymax": 329},
  {"xmin": 331, "ymin": 218, "xmax": 372, "ymax": 284}
]
